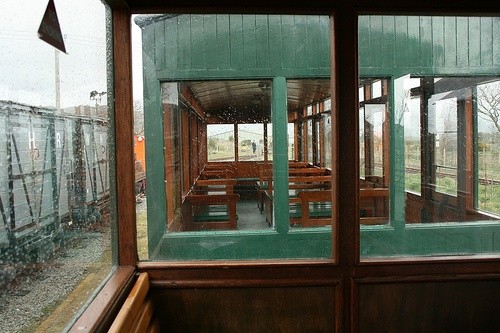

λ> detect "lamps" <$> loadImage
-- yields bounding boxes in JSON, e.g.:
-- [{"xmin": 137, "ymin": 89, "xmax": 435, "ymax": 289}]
[{"xmin": 258, "ymin": 79, "xmax": 271, "ymax": 95}]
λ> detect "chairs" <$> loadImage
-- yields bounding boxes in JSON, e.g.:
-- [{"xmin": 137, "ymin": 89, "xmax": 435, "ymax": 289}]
[{"xmin": 183, "ymin": 159, "xmax": 238, "ymax": 230}]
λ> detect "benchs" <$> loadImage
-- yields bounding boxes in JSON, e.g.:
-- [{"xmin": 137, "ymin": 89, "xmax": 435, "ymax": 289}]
[{"xmin": 254, "ymin": 162, "xmax": 390, "ymax": 228}]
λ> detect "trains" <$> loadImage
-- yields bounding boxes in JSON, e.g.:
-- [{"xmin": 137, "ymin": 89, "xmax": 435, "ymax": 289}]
[
  {"xmin": 0, "ymin": 100, "xmax": 108, "ymax": 293},
  {"xmin": 134, "ymin": 0, "xmax": 499, "ymax": 262}
]
